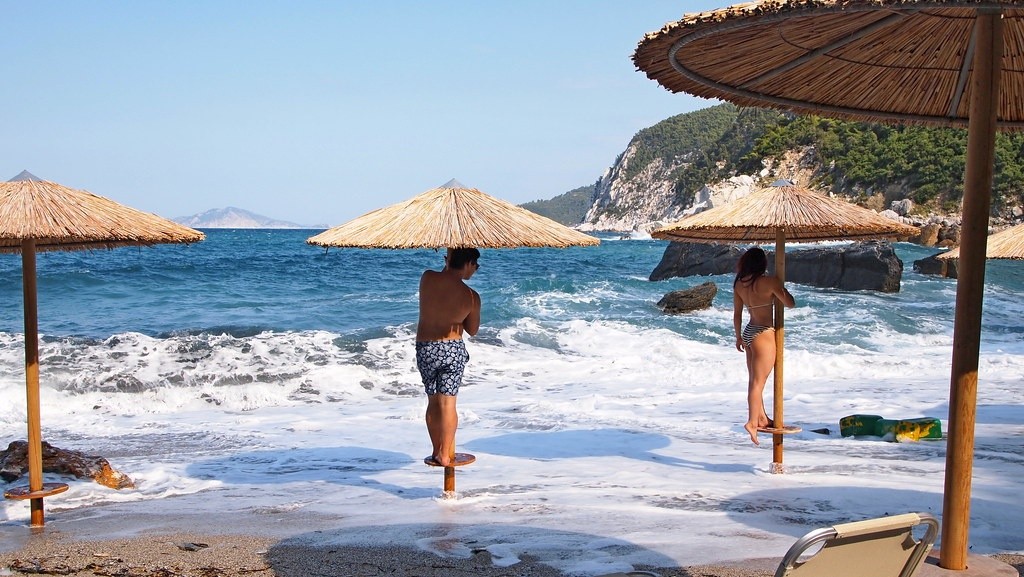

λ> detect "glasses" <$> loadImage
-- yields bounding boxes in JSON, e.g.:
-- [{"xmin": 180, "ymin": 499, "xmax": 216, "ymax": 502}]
[{"xmin": 473, "ymin": 261, "xmax": 480, "ymax": 270}]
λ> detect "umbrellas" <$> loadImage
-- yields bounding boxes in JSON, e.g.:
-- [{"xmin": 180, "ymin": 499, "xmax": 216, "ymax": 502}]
[
  {"xmin": 305, "ymin": 178, "xmax": 599, "ymax": 498},
  {"xmin": 650, "ymin": 176, "xmax": 922, "ymax": 463},
  {"xmin": 633, "ymin": 1, "xmax": 1024, "ymax": 570},
  {"xmin": 0, "ymin": 170, "xmax": 205, "ymax": 526}
]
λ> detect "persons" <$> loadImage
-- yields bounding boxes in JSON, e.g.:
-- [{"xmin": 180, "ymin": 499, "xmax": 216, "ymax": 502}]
[
  {"xmin": 416, "ymin": 247, "xmax": 482, "ymax": 467},
  {"xmin": 733, "ymin": 247, "xmax": 795, "ymax": 446}
]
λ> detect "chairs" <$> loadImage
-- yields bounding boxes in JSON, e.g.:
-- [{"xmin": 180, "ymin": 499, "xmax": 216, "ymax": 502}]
[{"xmin": 775, "ymin": 510, "xmax": 939, "ymax": 577}]
